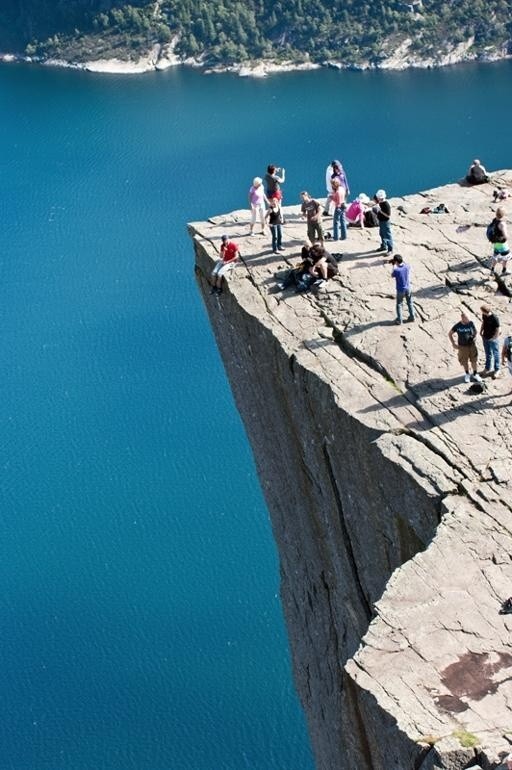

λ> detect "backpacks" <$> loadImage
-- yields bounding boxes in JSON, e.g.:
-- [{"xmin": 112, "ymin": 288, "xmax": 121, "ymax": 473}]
[{"xmin": 486, "ymin": 219, "xmax": 500, "ymax": 243}]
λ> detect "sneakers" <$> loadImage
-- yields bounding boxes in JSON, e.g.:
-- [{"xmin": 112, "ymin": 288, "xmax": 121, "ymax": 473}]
[
  {"xmin": 465, "ymin": 368, "xmax": 500, "ymax": 383},
  {"xmin": 376, "ymin": 247, "xmax": 393, "ymax": 256},
  {"xmin": 396, "ymin": 317, "xmax": 414, "ymax": 324},
  {"xmin": 209, "ymin": 285, "xmax": 223, "ymax": 297},
  {"xmin": 313, "ymin": 278, "xmax": 327, "ymax": 288}
]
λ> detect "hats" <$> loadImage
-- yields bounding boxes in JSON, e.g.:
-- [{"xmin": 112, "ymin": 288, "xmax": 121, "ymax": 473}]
[
  {"xmin": 253, "ymin": 177, "xmax": 262, "ymax": 187},
  {"xmin": 376, "ymin": 189, "xmax": 387, "ymax": 198},
  {"xmin": 222, "ymin": 235, "xmax": 228, "ymax": 240},
  {"xmin": 272, "ymin": 192, "xmax": 281, "ymax": 200},
  {"xmin": 356, "ymin": 193, "xmax": 369, "ymax": 204}
]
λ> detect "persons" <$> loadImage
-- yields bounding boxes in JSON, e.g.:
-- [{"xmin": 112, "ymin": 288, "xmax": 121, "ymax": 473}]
[
  {"xmin": 489, "ymin": 208, "xmax": 512, "ymax": 276},
  {"xmin": 500, "ymin": 336, "xmax": 512, "ymax": 375},
  {"xmin": 208, "ymin": 235, "xmax": 239, "ymax": 297},
  {"xmin": 465, "ymin": 159, "xmax": 488, "ymax": 185},
  {"xmin": 479, "ymin": 305, "xmax": 501, "ymax": 380},
  {"xmin": 391, "ymin": 255, "xmax": 415, "ymax": 324},
  {"xmin": 448, "ymin": 312, "xmax": 483, "ymax": 383},
  {"xmin": 248, "ymin": 159, "xmax": 393, "ymax": 292}
]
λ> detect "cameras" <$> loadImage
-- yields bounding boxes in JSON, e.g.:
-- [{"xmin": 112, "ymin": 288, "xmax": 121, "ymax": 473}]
[{"xmin": 383, "ymin": 259, "xmax": 394, "ymax": 265}]
